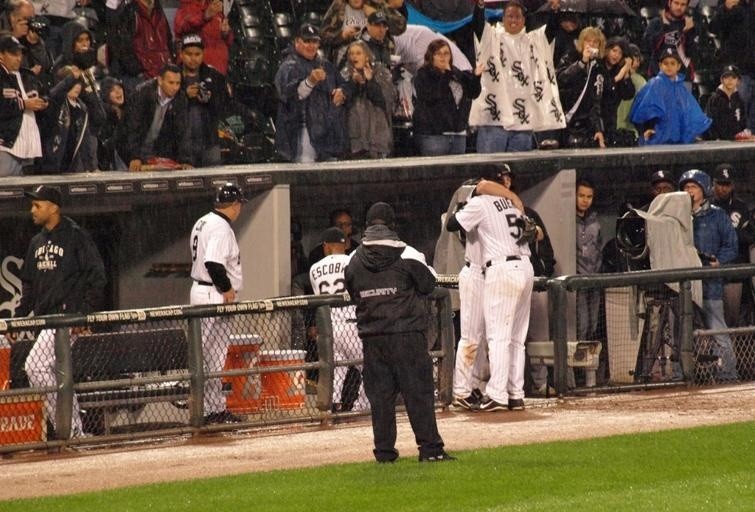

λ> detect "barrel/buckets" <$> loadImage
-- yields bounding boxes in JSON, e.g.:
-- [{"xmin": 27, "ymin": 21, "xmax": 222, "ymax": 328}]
[
  {"xmin": 221, "ymin": 330, "xmax": 262, "ymax": 410},
  {"xmin": 1, "ymin": 333, "xmax": 14, "ymax": 393},
  {"xmin": 260, "ymin": 348, "xmax": 308, "ymax": 411},
  {"xmin": 1, "ymin": 391, "xmax": 49, "ymax": 454}
]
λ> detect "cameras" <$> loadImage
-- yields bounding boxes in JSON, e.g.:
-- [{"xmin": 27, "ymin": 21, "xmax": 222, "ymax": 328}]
[
  {"xmin": 588, "ymin": 47, "xmax": 600, "ymax": 58},
  {"xmin": 196, "ymin": 81, "xmax": 210, "ymax": 103}
]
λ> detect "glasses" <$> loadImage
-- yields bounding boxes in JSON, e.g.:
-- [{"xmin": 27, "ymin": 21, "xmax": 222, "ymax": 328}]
[{"xmin": 434, "ymin": 52, "xmax": 450, "ymax": 56}]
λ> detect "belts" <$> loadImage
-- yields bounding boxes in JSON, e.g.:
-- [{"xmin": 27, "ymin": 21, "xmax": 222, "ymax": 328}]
[
  {"xmin": 487, "ymin": 255, "xmax": 521, "ymax": 267},
  {"xmin": 198, "ymin": 280, "xmax": 214, "ymax": 286},
  {"xmin": 465, "ymin": 261, "xmax": 470, "ymax": 267}
]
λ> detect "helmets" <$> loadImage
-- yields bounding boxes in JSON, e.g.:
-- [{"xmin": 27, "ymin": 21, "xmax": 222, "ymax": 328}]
[
  {"xmin": 678, "ymin": 170, "xmax": 712, "ymax": 198},
  {"xmin": 472, "ymin": 165, "xmax": 498, "ymax": 181},
  {"xmin": 216, "ymin": 183, "xmax": 248, "ymax": 203},
  {"xmin": 496, "ymin": 164, "xmax": 511, "ymax": 179}
]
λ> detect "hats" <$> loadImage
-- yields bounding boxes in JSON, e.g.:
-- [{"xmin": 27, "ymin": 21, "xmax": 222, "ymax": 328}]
[
  {"xmin": 367, "ymin": 203, "xmax": 396, "ymax": 227},
  {"xmin": 659, "ymin": 47, "xmax": 678, "ymax": 62},
  {"xmin": 182, "ymin": 33, "xmax": 203, "ymax": 51},
  {"xmin": 712, "ymin": 164, "xmax": 735, "ymax": 183},
  {"xmin": 0, "ymin": 34, "xmax": 25, "ymax": 52},
  {"xmin": 368, "ymin": 10, "xmax": 388, "ymax": 26},
  {"xmin": 721, "ymin": 65, "xmax": 741, "ymax": 79},
  {"xmin": 651, "ymin": 170, "xmax": 675, "ymax": 185},
  {"xmin": 23, "ymin": 185, "xmax": 63, "ymax": 207},
  {"xmin": 317, "ymin": 227, "xmax": 346, "ymax": 248},
  {"xmin": 298, "ymin": 22, "xmax": 320, "ymax": 42}
]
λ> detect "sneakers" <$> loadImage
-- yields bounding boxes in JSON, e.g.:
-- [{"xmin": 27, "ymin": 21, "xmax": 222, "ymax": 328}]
[
  {"xmin": 532, "ymin": 384, "xmax": 556, "ymax": 395},
  {"xmin": 419, "ymin": 452, "xmax": 458, "ymax": 461},
  {"xmin": 206, "ymin": 409, "xmax": 242, "ymax": 424},
  {"xmin": 452, "ymin": 387, "xmax": 525, "ymax": 411}
]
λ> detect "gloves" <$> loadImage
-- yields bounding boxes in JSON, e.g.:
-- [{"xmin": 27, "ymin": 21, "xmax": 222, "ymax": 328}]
[{"xmin": 521, "ymin": 215, "xmax": 536, "ymax": 240}]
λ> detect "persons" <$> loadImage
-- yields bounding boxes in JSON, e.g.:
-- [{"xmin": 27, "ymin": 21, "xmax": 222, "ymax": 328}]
[
  {"xmin": 187, "ymin": 183, "xmax": 249, "ymax": 431},
  {"xmin": 343, "ymin": 200, "xmax": 460, "ymax": 462},
  {"xmin": 292, "ymin": 162, "xmax": 754, "ymax": 423},
  {"xmin": 2, "ymin": 0, "xmax": 754, "ymax": 176},
  {"xmin": 2, "ymin": 182, "xmax": 110, "ymax": 452}
]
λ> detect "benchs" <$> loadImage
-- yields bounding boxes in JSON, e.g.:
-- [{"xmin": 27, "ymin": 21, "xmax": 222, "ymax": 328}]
[{"xmin": 9, "ymin": 328, "xmax": 232, "ymax": 436}]
